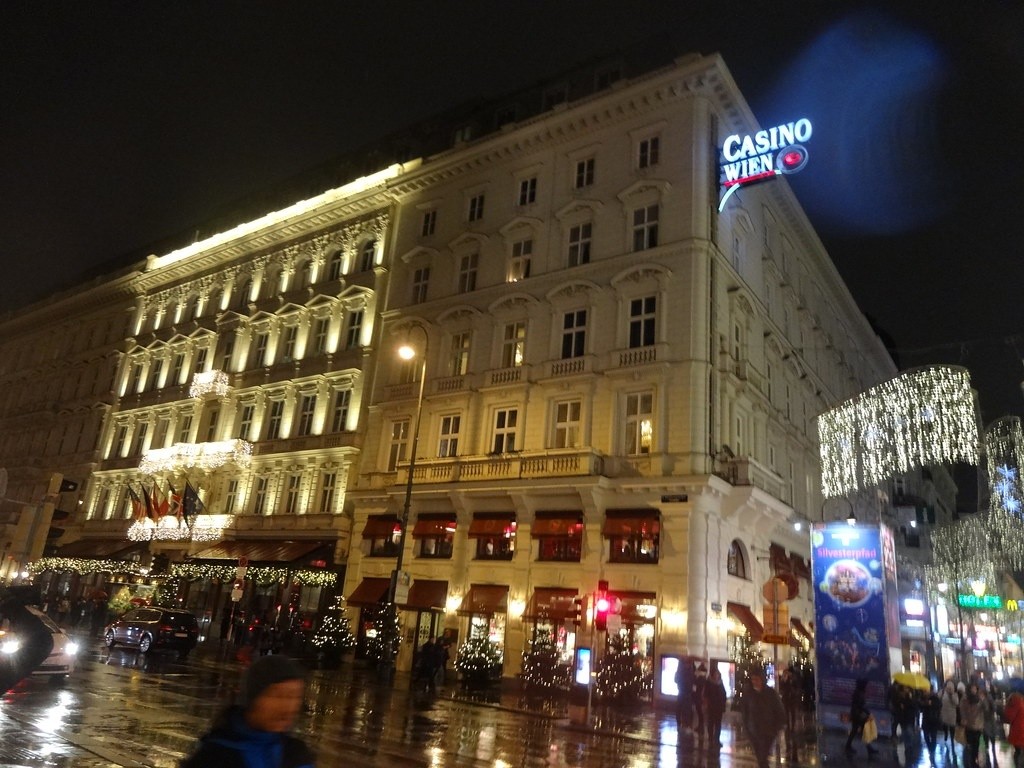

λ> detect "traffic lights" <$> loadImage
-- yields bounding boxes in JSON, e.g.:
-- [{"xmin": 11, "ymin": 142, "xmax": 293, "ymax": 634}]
[{"xmin": 594, "ymin": 581, "xmax": 611, "ymax": 629}]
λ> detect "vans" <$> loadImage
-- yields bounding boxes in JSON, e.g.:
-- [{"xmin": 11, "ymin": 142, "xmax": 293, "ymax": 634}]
[{"xmin": 103, "ymin": 605, "xmax": 200, "ymax": 655}]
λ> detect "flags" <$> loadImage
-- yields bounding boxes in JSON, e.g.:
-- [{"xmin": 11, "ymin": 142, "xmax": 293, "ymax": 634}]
[
  {"xmin": 128, "ymin": 488, "xmax": 145, "ymax": 521},
  {"xmin": 181, "ymin": 481, "xmax": 203, "ymax": 529},
  {"xmin": 141, "ymin": 486, "xmax": 159, "ymax": 523},
  {"xmin": 167, "ymin": 483, "xmax": 182, "ymax": 526},
  {"xmin": 151, "ymin": 483, "xmax": 169, "ymax": 517}
]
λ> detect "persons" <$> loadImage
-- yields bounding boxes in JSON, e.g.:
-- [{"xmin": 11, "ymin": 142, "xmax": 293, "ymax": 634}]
[
  {"xmin": 434, "ymin": 627, "xmax": 452, "ymax": 688},
  {"xmin": 417, "ymin": 636, "xmax": 441, "ymax": 693},
  {"xmin": 49, "ymin": 592, "xmax": 107, "ymax": 637},
  {"xmin": 780, "ymin": 659, "xmax": 815, "ymax": 730},
  {"xmin": 673, "ymin": 655, "xmax": 707, "ymax": 735},
  {"xmin": 845, "ymin": 678, "xmax": 879, "ymax": 754},
  {"xmin": 886, "ymin": 678, "xmax": 1006, "ymax": 768},
  {"xmin": 702, "ymin": 669, "xmax": 727, "ymax": 747},
  {"xmin": 740, "ymin": 668, "xmax": 787, "ymax": 768},
  {"xmin": 176, "ymin": 656, "xmax": 316, "ymax": 768},
  {"xmin": 259, "ymin": 624, "xmax": 285, "ymax": 656},
  {"xmin": 1002, "ymin": 677, "xmax": 1024, "ymax": 768}
]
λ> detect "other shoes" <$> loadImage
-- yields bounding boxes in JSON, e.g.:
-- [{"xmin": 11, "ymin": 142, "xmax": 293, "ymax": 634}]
[{"xmin": 694, "ymin": 726, "xmax": 723, "ymax": 747}]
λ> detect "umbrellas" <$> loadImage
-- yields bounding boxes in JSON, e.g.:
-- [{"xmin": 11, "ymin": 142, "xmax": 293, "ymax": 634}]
[
  {"xmin": 891, "ymin": 670, "xmax": 932, "ymax": 693},
  {"xmin": 87, "ymin": 590, "xmax": 107, "ymax": 600}
]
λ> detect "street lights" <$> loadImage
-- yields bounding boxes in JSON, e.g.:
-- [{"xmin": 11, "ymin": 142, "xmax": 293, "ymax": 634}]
[{"xmin": 385, "ymin": 319, "xmax": 431, "ymax": 606}]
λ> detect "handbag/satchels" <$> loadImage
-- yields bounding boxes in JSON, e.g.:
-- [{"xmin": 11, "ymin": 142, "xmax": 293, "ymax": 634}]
[
  {"xmin": 863, "ymin": 720, "xmax": 877, "ymax": 744},
  {"xmin": 955, "ymin": 724, "xmax": 967, "ymax": 744},
  {"xmin": 975, "ymin": 734, "xmax": 987, "ymax": 767}
]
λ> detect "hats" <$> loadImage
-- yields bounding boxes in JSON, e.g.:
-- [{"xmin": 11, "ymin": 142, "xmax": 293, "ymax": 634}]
[
  {"xmin": 957, "ymin": 682, "xmax": 965, "ymax": 692},
  {"xmin": 246, "ymin": 655, "xmax": 304, "ymax": 694}
]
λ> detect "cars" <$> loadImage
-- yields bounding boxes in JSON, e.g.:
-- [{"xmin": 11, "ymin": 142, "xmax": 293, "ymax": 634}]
[{"xmin": 0, "ymin": 604, "xmax": 79, "ymax": 678}]
